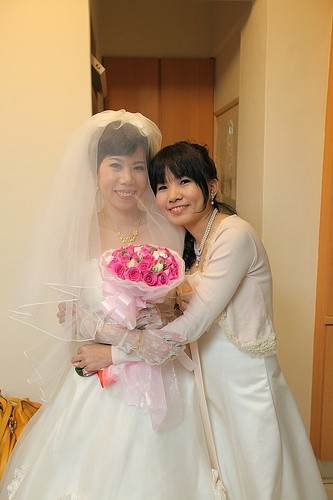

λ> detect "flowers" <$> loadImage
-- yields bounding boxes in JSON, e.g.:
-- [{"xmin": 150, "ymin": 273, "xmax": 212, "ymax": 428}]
[{"xmin": 98, "ymin": 244, "xmax": 186, "ymax": 328}]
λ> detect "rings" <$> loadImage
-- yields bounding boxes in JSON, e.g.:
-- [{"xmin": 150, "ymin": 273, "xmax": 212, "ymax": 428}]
[
  {"xmin": 82, "ymin": 368, "xmax": 87, "ymax": 374},
  {"xmin": 164, "ymin": 354, "xmax": 172, "ymax": 361}
]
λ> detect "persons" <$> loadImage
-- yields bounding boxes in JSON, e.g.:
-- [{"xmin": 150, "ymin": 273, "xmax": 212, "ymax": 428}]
[
  {"xmin": 0, "ymin": 108, "xmax": 229, "ymax": 500},
  {"xmin": 57, "ymin": 141, "xmax": 333, "ymax": 500}
]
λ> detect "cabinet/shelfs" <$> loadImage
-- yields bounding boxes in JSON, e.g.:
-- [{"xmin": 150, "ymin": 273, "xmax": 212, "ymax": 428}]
[{"xmin": 102, "ymin": 55, "xmax": 215, "ymax": 163}]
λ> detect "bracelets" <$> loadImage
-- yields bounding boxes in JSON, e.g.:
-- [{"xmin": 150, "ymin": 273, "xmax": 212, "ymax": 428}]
[{"xmin": 135, "ymin": 329, "xmax": 142, "ymax": 353}]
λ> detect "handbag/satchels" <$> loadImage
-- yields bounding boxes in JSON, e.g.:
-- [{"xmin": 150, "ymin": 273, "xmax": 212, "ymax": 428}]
[{"xmin": 0, "ymin": 390, "xmax": 42, "ymax": 482}]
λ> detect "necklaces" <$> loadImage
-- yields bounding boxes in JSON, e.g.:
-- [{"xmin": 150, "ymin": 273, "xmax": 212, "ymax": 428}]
[
  {"xmin": 194, "ymin": 208, "xmax": 218, "ymax": 267},
  {"xmin": 100, "ymin": 208, "xmax": 143, "ymax": 247}
]
